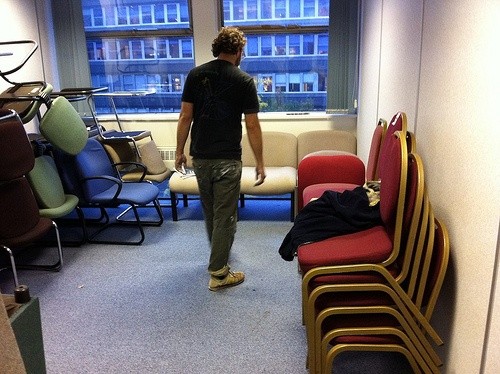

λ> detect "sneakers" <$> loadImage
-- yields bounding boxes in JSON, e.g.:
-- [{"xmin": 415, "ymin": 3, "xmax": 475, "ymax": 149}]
[{"xmin": 208, "ymin": 270, "xmax": 245, "ymax": 291}]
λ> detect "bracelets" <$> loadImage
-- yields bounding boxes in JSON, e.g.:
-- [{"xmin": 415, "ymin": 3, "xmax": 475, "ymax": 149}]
[{"xmin": 175, "ymin": 151, "xmax": 184, "ymax": 154}]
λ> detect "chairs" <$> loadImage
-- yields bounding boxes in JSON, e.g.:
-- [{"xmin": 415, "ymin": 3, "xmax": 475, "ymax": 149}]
[
  {"xmin": 0, "ymin": 39, "xmax": 172, "ymax": 287},
  {"xmin": 296, "ymin": 130, "xmax": 357, "ymax": 215},
  {"xmin": 295, "ymin": 111, "xmax": 451, "ymax": 374},
  {"xmin": 169, "ymin": 128, "xmax": 201, "ymax": 222},
  {"xmin": 238, "ymin": 129, "xmax": 298, "ymax": 222}
]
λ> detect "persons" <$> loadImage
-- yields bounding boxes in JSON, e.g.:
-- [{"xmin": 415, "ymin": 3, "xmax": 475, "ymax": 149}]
[{"xmin": 174, "ymin": 26, "xmax": 267, "ymax": 292}]
